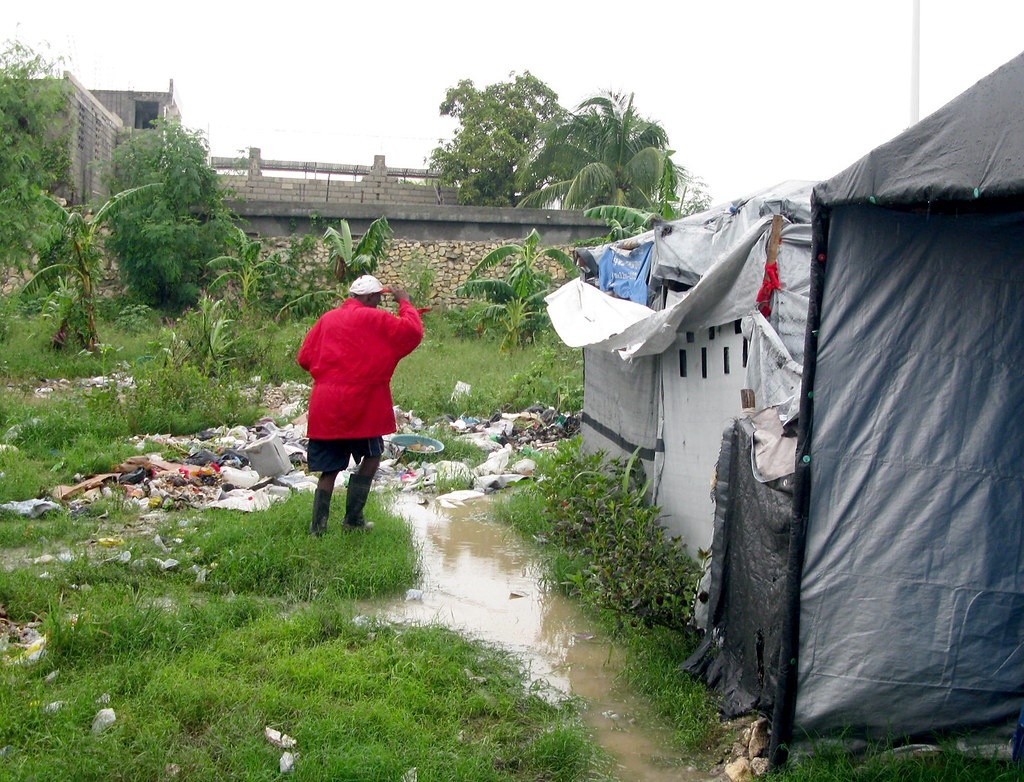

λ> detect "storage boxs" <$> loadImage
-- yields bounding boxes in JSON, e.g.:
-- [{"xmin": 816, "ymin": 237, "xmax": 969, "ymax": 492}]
[{"xmin": 244, "ymin": 432, "xmax": 293, "ymax": 478}]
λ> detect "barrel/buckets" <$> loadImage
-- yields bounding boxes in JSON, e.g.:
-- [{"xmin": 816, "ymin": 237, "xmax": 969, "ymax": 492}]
[{"xmin": 245, "ymin": 434, "xmax": 292, "ymax": 478}]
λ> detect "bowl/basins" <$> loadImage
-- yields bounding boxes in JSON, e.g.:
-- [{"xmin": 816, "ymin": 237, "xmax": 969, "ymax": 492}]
[{"xmin": 391, "ymin": 434, "xmax": 445, "ymax": 462}]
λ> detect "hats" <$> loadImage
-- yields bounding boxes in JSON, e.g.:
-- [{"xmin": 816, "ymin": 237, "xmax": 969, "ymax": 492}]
[{"xmin": 349, "ymin": 274, "xmax": 392, "ymax": 295}]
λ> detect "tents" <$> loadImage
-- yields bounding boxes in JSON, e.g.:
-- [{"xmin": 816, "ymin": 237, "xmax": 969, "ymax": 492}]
[
  {"xmin": 542, "ymin": 179, "xmax": 810, "ymax": 633},
  {"xmin": 769, "ymin": 54, "xmax": 1024, "ymax": 782}
]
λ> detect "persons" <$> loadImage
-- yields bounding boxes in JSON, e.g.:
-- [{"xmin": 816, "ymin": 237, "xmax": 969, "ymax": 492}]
[{"xmin": 296, "ymin": 275, "xmax": 424, "ymax": 539}]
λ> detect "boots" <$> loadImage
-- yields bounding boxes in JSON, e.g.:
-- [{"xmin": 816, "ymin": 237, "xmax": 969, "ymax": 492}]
[
  {"xmin": 309, "ymin": 488, "xmax": 331, "ymax": 537},
  {"xmin": 342, "ymin": 473, "xmax": 374, "ymax": 532}
]
